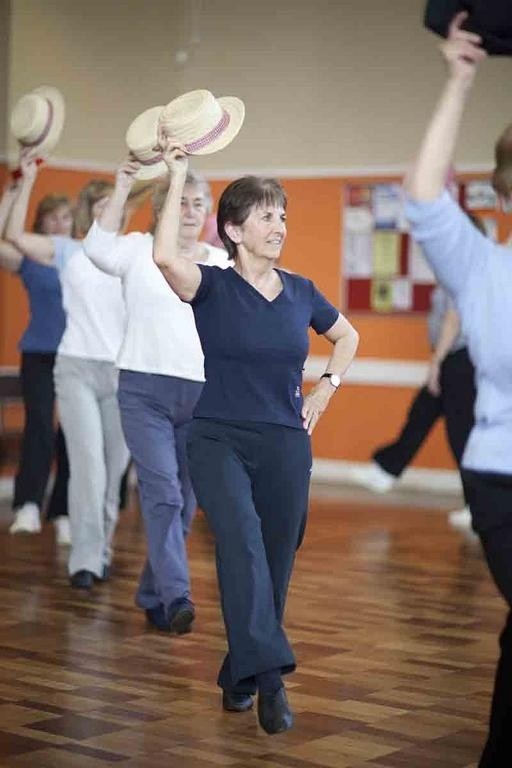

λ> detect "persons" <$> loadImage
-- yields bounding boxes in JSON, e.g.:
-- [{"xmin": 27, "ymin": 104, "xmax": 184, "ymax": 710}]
[
  {"xmin": 82, "ymin": 152, "xmax": 235, "ymax": 632},
  {"xmin": 3, "ymin": 145, "xmax": 134, "ymax": 589},
  {"xmin": 393, "ymin": 11, "xmax": 512, "ymax": 768},
  {"xmin": 0, "ymin": 176, "xmax": 76, "ymax": 547},
  {"xmin": 352, "ymin": 211, "xmax": 487, "ymax": 533},
  {"xmin": 153, "ymin": 140, "xmax": 359, "ymax": 735}
]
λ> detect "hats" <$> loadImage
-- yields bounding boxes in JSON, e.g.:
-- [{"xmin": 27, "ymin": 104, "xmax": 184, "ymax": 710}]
[
  {"xmin": 126, "ymin": 106, "xmax": 168, "ymax": 179},
  {"xmin": 424, "ymin": 0, "xmax": 512, "ymax": 58},
  {"xmin": 10, "ymin": 85, "xmax": 64, "ymax": 155},
  {"xmin": 158, "ymin": 89, "xmax": 245, "ymax": 156}
]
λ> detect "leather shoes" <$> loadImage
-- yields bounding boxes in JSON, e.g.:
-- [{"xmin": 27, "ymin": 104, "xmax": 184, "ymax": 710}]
[
  {"xmin": 147, "ymin": 605, "xmax": 170, "ymax": 631},
  {"xmin": 258, "ymin": 684, "xmax": 292, "ymax": 735},
  {"xmin": 71, "ymin": 571, "xmax": 93, "ymax": 587},
  {"xmin": 169, "ymin": 598, "xmax": 195, "ymax": 635},
  {"xmin": 221, "ymin": 690, "xmax": 252, "ymax": 711},
  {"xmin": 94, "ymin": 565, "xmax": 110, "ymax": 582}
]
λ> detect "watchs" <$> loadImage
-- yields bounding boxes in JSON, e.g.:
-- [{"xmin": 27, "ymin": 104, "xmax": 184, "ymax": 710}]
[{"xmin": 322, "ymin": 373, "xmax": 340, "ymax": 388}]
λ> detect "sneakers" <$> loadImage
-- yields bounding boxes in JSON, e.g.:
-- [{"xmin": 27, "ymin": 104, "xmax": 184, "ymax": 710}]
[
  {"xmin": 54, "ymin": 517, "xmax": 71, "ymax": 546},
  {"xmin": 11, "ymin": 506, "xmax": 42, "ymax": 534},
  {"xmin": 348, "ymin": 461, "xmax": 394, "ymax": 494},
  {"xmin": 448, "ymin": 507, "xmax": 471, "ymax": 530}
]
